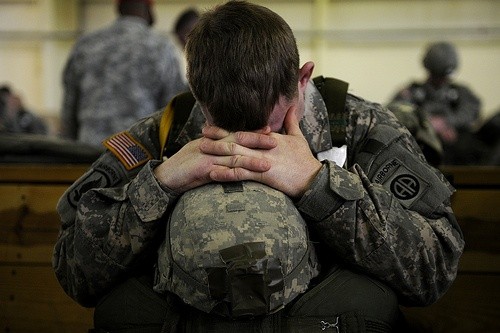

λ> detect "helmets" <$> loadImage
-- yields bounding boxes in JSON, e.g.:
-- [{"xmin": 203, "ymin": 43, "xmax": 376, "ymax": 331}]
[
  {"xmin": 423, "ymin": 43, "xmax": 457, "ymax": 74},
  {"xmin": 158, "ymin": 182, "xmax": 318, "ymax": 317}
]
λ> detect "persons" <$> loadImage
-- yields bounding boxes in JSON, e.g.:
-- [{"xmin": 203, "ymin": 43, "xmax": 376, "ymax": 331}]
[
  {"xmin": 389, "ymin": 41, "xmax": 487, "ymax": 160},
  {"xmin": 174, "ymin": 9, "xmax": 204, "ymax": 51},
  {"xmin": 62, "ymin": 0, "xmax": 189, "ymax": 144},
  {"xmin": 0, "ymin": 84, "xmax": 48, "ymax": 136},
  {"xmin": 51, "ymin": 0, "xmax": 467, "ymax": 333}
]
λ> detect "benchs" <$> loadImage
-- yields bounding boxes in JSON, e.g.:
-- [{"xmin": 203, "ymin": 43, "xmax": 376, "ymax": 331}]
[{"xmin": 1, "ymin": 164, "xmax": 499, "ymax": 333}]
